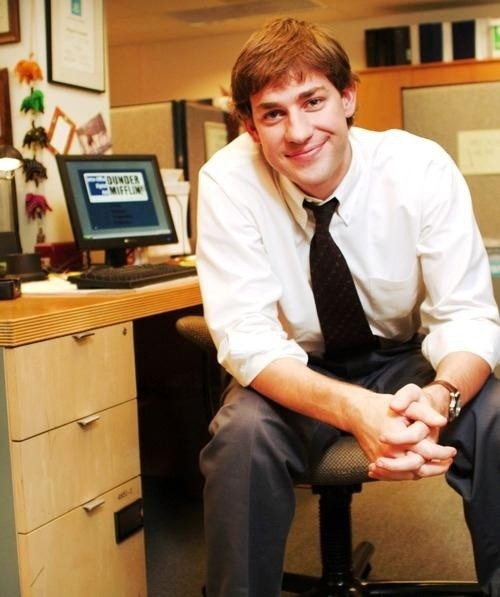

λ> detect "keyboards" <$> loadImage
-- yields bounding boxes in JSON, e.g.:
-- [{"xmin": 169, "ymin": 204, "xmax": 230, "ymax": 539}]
[{"xmin": 66, "ymin": 262, "xmax": 199, "ymax": 289}]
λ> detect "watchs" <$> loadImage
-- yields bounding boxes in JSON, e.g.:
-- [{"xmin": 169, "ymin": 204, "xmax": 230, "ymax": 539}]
[{"xmin": 427, "ymin": 380, "xmax": 462, "ymax": 423}]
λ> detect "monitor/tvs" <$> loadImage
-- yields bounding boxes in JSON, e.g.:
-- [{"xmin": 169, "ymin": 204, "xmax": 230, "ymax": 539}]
[{"xmin": 55, "ymin": 153, "xmax": 179, "ymax": 267}]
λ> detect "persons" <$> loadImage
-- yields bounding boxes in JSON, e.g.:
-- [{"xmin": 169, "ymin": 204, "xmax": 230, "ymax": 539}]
[{"xmin": 194, "ymin": 17, "xmax": 500, "ymax": 595}]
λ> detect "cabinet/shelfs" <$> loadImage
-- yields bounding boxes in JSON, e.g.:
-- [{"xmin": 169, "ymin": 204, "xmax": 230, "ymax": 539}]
[{"xmin": 356, "ymin": 58, "xmax": 499, "ymax": 307}]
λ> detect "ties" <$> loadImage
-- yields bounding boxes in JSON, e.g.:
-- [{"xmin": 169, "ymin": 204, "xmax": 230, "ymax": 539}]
[{"xmin": 303, "ymin": 198, "xmax": 374, "ymax": 378}]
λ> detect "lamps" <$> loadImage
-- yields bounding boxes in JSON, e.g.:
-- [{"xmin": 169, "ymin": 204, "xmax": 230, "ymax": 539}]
[{"xmin": 0, "ymin": 138, "xmax": 24, "ymax": 170}]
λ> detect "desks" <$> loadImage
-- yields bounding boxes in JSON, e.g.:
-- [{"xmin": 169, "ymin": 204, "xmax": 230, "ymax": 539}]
[{"xmin": 0, "ymin": 255, "xmax": 204, "ymax": 597}]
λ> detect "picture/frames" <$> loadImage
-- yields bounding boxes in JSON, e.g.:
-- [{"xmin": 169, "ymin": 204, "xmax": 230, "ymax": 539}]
[
  {"xmin": 0, "ymin": 0, "xmax": 21, "ymax": 47},
  {"xmin": 46, "ymin": 107, "xmax": 75, "ymax": 157}
]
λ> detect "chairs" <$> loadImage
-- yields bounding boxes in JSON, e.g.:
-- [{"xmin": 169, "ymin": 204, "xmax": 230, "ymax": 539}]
[{"xmin": 172, "ymin": 302, "xmax": 491, "ymax": 597}]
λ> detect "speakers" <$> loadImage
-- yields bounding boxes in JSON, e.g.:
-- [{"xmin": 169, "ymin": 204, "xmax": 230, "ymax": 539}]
[
  {"xmin": 363, "ymin": 24, "xmax": 412, "ymax": 68},
  {"xmin": 451, "ymin": 19, "xmax": 476, "ymax": 60},
  {"xmin": 419, "ymin": 22, "xmax": 443, "ymax": 63}
]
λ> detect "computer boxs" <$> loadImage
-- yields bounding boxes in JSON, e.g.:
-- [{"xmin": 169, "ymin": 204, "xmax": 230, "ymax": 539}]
[{"xmin": 110, "ymin": 98, "xmax": 240, "ymax": 259}]
